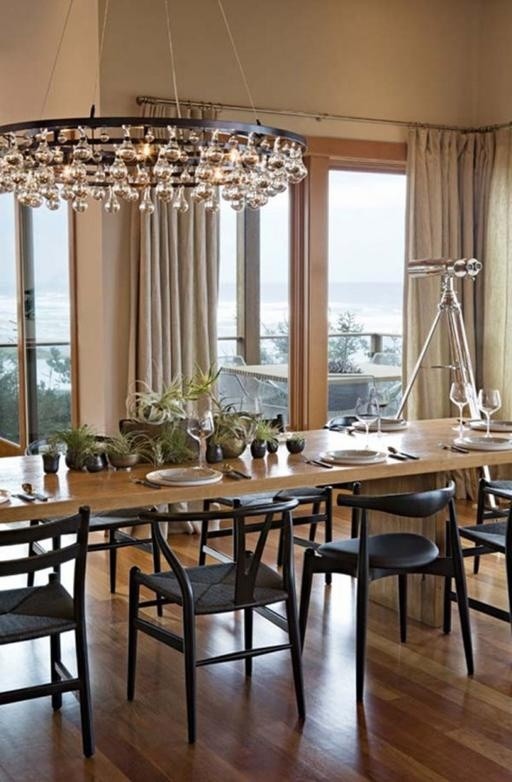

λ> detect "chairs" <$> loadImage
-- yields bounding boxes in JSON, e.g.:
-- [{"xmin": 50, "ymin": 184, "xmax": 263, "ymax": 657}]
[
  {"xmin": 215, "ymin": 354, "xmax": 290, "ymax": 426},
  {"xmin": 0, "ymin": 409, "xmax": 511, "ymax": 759},
  {"xmin": 351, "ymin": 350, "xmax": 404, "ymax": 420}
]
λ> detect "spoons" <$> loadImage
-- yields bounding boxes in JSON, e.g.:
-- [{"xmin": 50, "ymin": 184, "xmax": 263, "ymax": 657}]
[
  {"xmin": 221, "ymin": 462, "xmax": 253, "ymax": 481},
  {"xmin": 22, "ymin": 481, "xmax": 48, "ymax": 502},
  {"xmin": 387, "ymin": 446, "xmax": 420, "ymax": 461}
]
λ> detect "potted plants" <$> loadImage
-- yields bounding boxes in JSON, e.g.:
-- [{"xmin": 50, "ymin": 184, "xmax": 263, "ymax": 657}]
[{"xmin": 118, "ymin": 361, "xmax": 222, "ymax": 462}]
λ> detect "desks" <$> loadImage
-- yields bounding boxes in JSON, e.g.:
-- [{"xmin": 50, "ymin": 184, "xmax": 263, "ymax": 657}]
[{"xmin": 217, "ymin": 361, "xmax": 403, "ymax": 407}]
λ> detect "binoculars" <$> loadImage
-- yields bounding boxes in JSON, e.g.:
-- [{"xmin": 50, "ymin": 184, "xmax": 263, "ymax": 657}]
[{"xmin": 407, "ymin": 257, "xmax": 482, "ymax": 280}]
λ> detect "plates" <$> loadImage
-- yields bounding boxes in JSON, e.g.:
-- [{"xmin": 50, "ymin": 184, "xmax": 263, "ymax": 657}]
[
  {"xmin": 1, "ymin": 489, "xmax": 12, "ymax": 507},
  {"xmin": 452, "ymin": 436, "xmax": 512, "ymax": 452},
  {"xmin": 146, "ymin": 466, "xmax": 225, "ymax": 486},
  {"xmin": 319, "ymin": 448, "xmax": 389, "ymax": 465},
  {"xmin": 465, "ymin": 419, "xmax": 512, "ymax": 433},
  {"xmin": 352, "ymin": 417, "xmax": 410, "ymax": 431}
]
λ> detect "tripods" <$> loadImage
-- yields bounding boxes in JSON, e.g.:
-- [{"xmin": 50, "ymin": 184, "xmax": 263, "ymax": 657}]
[{"xmin": 395, "ymin": 274, "xmax": 498, "ymax": 507}]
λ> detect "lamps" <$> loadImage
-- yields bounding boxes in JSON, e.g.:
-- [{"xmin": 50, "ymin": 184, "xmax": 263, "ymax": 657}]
[{"xmin": 1, "ymin": 2, "xmax": 311, "ymax": 216}]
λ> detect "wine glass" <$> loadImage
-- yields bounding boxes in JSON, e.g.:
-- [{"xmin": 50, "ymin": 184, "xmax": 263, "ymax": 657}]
[
  {"xmin": 185, "ymin": 408, "xmax": 216, "ymax": 472},
  {"xmin": 476, "ymin": 388, "xmax": 502, "ymax": 438},
  {"xmin": 353, "ymin": 383, "xmax": 390, "ymax": 446},
  {"xmin": 449, "ymin": 381, "xmax": 474, "ymax": 433}
]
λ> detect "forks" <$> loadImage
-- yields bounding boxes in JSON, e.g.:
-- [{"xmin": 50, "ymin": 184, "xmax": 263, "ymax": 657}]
[
  {"xmin": 303, "ymin": 456, "xmax": 333, "ymax": 468},
  {"xmin": 128, "ymin": 472, "xmax": 162, "ymax": 490},
  {"xmin": 435, "ymin": 440, "xmax": 469, "ymax": 454}
]
